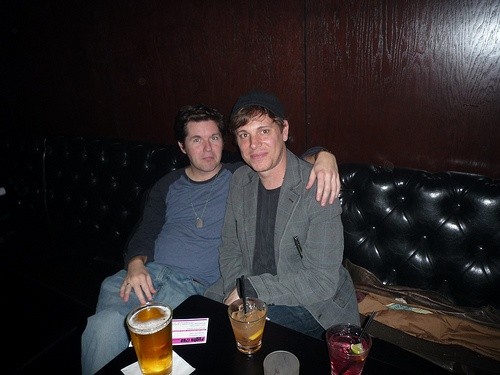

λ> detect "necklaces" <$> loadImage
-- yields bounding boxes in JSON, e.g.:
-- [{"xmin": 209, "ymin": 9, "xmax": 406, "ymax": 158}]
[{"xmin": 188, "ymin": 164, "xmax": 220, "ymax": 228}]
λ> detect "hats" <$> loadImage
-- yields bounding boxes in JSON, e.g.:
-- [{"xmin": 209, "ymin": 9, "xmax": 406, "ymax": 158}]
[{"xmin": 229, "ymin": 89, "xmax": 285, "ymax": 119}]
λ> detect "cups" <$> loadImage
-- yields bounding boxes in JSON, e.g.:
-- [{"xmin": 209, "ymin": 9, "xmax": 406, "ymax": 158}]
[
  {"xmin": 326, "ymin": 322, "xmax": 372, "ymax": 375},
  {"xmin": 227, "ymin": 296, "xmax": 268, "ymax": 354},
  {"xmin": 125, "ymin": 301, "xmax": 174, "ymax": 375},
  {"xmin": 263, "ymin": 350, "xmax": 300, "ymax": 375}
]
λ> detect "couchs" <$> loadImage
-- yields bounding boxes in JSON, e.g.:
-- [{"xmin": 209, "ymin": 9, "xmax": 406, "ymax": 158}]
[{"xmin": 0, "ymin": 133, "xmax": 500, "ymax": 375}]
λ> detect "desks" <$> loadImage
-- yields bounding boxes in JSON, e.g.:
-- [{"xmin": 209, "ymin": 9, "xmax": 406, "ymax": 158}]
[{"xmin": 95, "ymin": 295, "xmax": 420, "ymax": 375}]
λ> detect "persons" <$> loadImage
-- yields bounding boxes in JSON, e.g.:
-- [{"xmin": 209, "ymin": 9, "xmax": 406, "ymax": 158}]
[
  {"xmin": 204, "ymin": 93, "xmax": 362, "ymax": 338},
  {"xmin": 81, "ymin": 106, "xmax": 342, "ymax": 375}
]
